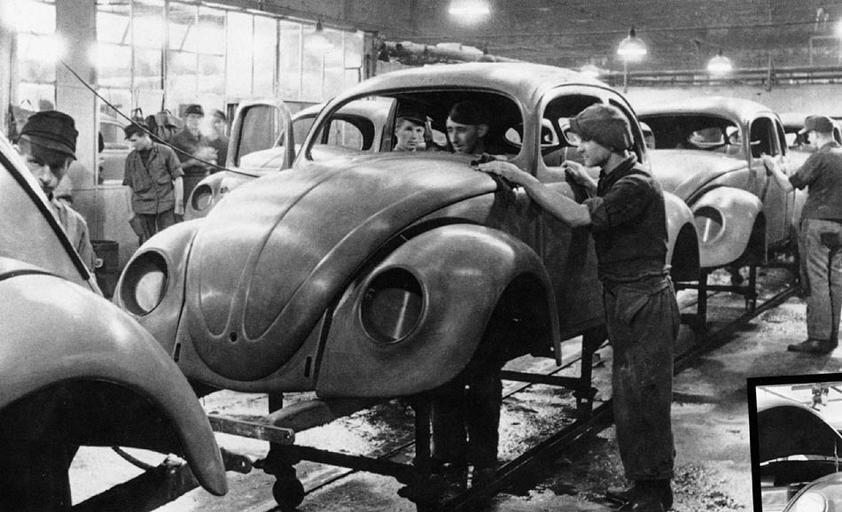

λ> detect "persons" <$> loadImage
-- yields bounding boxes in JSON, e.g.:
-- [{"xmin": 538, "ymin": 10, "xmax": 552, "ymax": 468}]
[
  {"xmin": 390, "ymin": 104, "xmax": 427, "ymax": 151},
  {"xmin": 760, "ymin": 116, "xmax": 842, "ymax": 354},
  {"xmin": 205, "ymin": 109, "xmax": 231, "ymax": 174},
  {"xmin": 425, "ymin": 103, "xmax": 517, "ymax": 484},
  {"xmin": 13, "ymin": 110, "xmax": 97, "ymax": 283},
  {"xmin": 170, "ymin": 106, "xmax": 219, "ymax": 204},
  {"xmin": 477, "ymin": 103, "xmax": 680, "ymax": 512},
  {"xmin": 124, "ymin": 123, "xmax": 184, "ymax": 244}
]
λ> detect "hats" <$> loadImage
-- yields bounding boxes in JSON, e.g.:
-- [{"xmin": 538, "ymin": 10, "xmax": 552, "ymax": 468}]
[
  {"xmin": 568, "ymin": 103, "xmax": 635, "ymax": 153},
  {"xmin": 448, "ymin": 99, "xmax": 492, "ymax": 126},
  {"xmin": 19, "ymin": 111, "xmax": 79, "ymax": 162},
  {"xmin": 209, "ymin": 108, "xmax": 227, "ymax": 125},
  {"xmin": 184, "ymin": 104, "xmax": 205, "ymax": 118},
  {"xmin": 797, "ymin": 113, "xmax": 835, "ymax": 135},
  {"xmin": 123, "ymin": 121, "xmax": 148, "ymax": 141},
  {"xmin": 396, "ymin": 103, "xmax": 429, "ymax": 126}
]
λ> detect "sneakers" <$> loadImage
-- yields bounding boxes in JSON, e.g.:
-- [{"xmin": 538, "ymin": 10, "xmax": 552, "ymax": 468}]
[
  {"xmin": 606, "ymin": 480, "xmax": 673, "ymax": 512},
  {"xmin": 787, "ymin": 332, "xmax": 838, "ymax": 356}
]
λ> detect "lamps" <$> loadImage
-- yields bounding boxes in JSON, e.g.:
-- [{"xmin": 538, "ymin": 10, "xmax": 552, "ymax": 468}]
[
  {"xmin": 617, "ymin": 26, "xmax": 647, "ymax": 56},
  {"xmin": 706, "ymin": 46, "xmax": 733, "ymax": 74},
  {"xmin": 306, "ymin": 19, "xmax": 330, "ymax": 46},
  {"xmin": 447, "ymin": 0, "xmax": 491, "ymax": 15}
]
